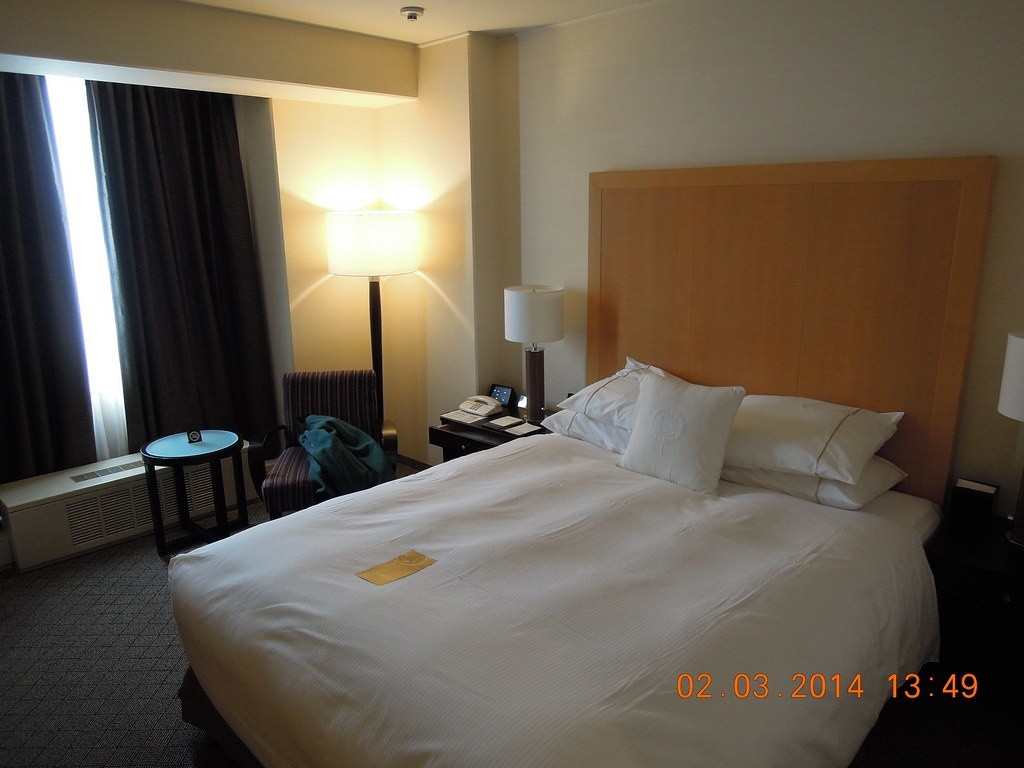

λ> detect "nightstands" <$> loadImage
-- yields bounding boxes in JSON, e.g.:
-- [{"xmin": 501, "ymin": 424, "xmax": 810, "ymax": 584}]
[
  {"xmin": 922, "ymin": 511, "xmax": 1024, "ymax": 681},
  {"xmin": 428, "ymin": 407, "xmax": 559, "ymax": 465}
]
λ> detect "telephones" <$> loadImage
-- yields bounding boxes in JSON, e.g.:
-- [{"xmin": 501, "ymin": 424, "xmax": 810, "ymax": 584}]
[{"xmin": 458, "ymin": 393, "xmax": 503, "ymax": 417}]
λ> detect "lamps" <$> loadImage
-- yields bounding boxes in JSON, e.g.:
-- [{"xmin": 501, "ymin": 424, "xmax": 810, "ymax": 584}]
[
  {"xmin": 987, "ymin": 332, "xmax": 1024, "ymax": 545},
  {"xmin": 326, "ymin": 211, "xmax": 428, "ymax": 455},
  {"xmin": 505, "ymin": 284, "xmax": 566, "ymax": 421}
]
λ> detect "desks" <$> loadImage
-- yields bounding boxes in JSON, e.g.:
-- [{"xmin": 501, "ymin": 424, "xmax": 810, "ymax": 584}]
[{"xmin": 140, "ymin": 429, "xmax": 249, "ymax": 558}]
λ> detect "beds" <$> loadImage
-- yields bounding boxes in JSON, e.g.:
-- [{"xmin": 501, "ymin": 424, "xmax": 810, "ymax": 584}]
[{"xmin": 167, "ymin": 429, "xmax": 940, "ymax": 757}]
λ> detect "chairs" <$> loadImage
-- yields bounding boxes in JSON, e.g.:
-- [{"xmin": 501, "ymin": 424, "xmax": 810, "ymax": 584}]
[{"xmin": 262, "ymin": 370, "xmax": 396, "ymax": 513}]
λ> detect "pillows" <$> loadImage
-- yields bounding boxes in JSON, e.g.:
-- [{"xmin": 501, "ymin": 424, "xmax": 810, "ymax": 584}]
[
  {"xmin": 557, "ymin": 357, "xmax": 690, "ymax": 430},
  {"xmin": 721, "ymin": 394, "xmax": 905, "ymax": 485},
  {"xmin": 540, "ymin": 410, "xmax": 633, "ymax": 459},
  {"xmin": 712, "ymin": 455, "xmax": 909, "ymax": 510},
  {"xmin": 616, "ymin": 373, "xmax": 746, "ymax": 496}
]
